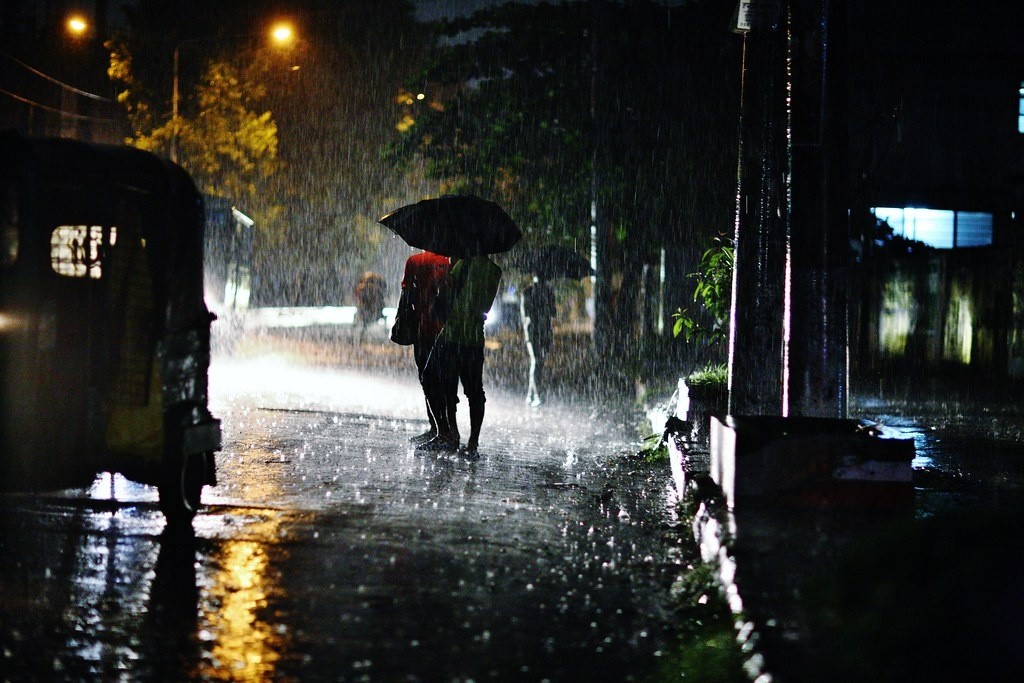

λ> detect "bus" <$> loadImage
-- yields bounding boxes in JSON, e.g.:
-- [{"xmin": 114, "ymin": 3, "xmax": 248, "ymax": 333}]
[{"xmin": 204, "ymin": 193, "xmax": 253, "ymax": 330}]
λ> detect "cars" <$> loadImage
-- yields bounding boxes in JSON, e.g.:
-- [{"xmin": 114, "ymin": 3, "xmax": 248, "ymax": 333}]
[{"xmin": 0, "ymin": 137, "xmax": 225, "ymax": 524}]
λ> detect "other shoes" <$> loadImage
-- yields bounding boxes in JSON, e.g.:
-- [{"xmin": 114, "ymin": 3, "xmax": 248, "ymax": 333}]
[
  {"xmin": 458, "ymin": 442, "xmax": 480, "ymax": 460},
  {"xmin": 524, "ymin": 394, "xmax": 545, "ymax": 407},
  {"xmin": 415, "ymin": 435, "xmax": 458, "ymax": 452},
  {"xmin": 408, "ymin": 429, "xmax": 461, "ymax": 449}
]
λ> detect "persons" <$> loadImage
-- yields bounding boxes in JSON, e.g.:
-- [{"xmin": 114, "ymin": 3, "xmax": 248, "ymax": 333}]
[
  {"xmin": 522, "ymin": 277, "xmax": 556, "ymax": 406},
  {"xmin": 613, "ymin": 274, "xmax": 639, "ymax": 350},
  {"xmin": 354, "ymin": 271, "xmax": 387, "ymax": 328},
  {"xmin": 388, "ymin": 242, "xmax": 504, "ymax": 459}
]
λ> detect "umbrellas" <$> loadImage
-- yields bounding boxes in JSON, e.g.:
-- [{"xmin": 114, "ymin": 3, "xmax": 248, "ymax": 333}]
[
  {"xmin": 510, "ymin": 246, "xmax": 600, "ymax": 280},
  {"xmin": 378, "ymin": 196, "xmax": 523, "ymax": 258}
]
